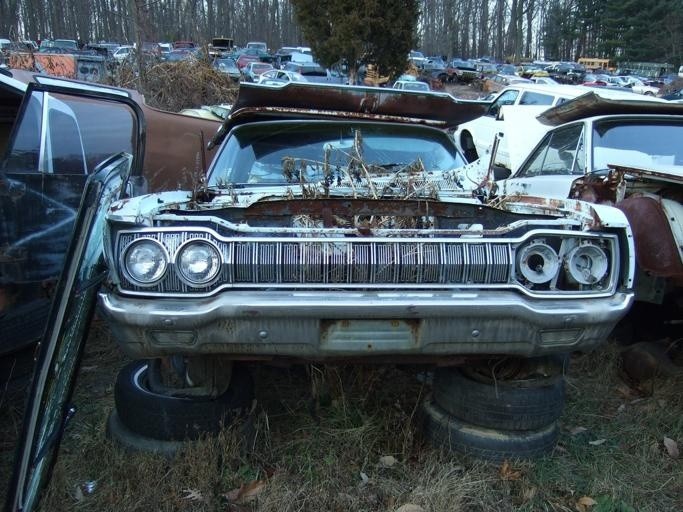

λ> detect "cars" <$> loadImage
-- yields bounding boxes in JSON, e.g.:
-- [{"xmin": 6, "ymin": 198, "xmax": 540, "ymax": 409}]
[{"xmin": 394, "ymin": 50, "xmax": 661, "ymax": 105}]
